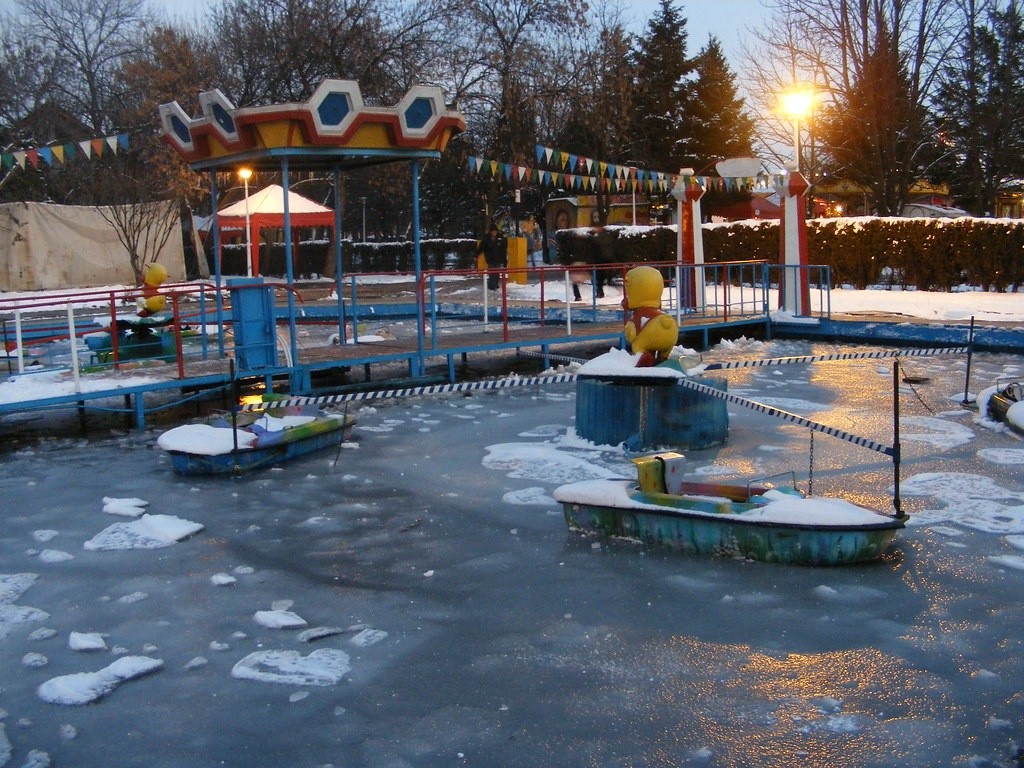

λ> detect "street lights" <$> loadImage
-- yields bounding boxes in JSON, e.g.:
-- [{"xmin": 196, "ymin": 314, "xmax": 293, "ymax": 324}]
[
  {"xmin": 361, "ymin": 196, "xmax": 367, "ymax": 243},
  {"xmin": 787, "ymin": 92, "xmax": 813, "ymax": 173},
  {"xmin": 240, "ymin": 169, "xmax": 253, "ymax": 279}
]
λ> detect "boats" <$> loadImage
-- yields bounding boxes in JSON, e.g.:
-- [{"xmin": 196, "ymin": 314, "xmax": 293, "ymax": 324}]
[
  {"xmin": 958, "ymin": 315, "xmax": 1024, "ymax": 438},
  {"xmin": 545, "ymin": 362, "xmax": 914, "ymax": 567},
  {"xmin": 157, "ymin": 360, "xmax": 357, "ymax": 476}
]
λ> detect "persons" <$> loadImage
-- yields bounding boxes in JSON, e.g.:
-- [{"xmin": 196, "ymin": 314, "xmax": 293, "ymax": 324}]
[
  {"xmin": 521, "ymin": 229, "xmax": 537, "ymax": 267},
  {"xmin": 558, "ymin": 227, "xmax": 616, "ymax": 300},
  {"xmin": 472, "ymin": 224, "xmax": 508, "ymax": 294}
]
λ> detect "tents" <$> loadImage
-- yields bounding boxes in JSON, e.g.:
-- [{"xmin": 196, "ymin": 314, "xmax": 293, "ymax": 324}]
[{"xmin": 216, "ymin": 184, "xmax": 337, "ymax": 276}]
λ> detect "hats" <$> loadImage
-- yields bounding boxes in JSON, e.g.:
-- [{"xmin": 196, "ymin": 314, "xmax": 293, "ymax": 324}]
[{"xmin": 488, "ymin": 224, "xmax": 497, "ymax": 231}]
